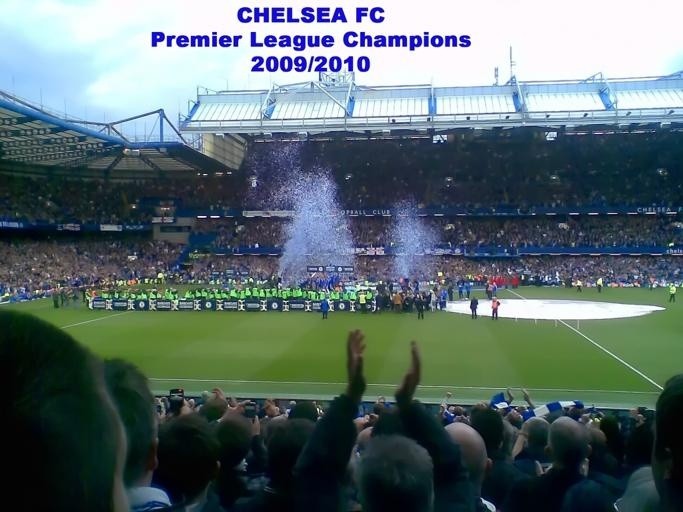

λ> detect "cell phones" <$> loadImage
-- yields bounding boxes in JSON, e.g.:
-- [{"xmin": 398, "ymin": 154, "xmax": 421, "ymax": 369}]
[
  {"xmin": 244, "ymin": 402, "xmax": 257, "ymax": 418},
  {"xmin": 170, "ymin": 389, "xmax": 184, "ymax": 405},
  {"xmin": 156, "ymin": 405, "xmax": 161, "ymax": 412}
]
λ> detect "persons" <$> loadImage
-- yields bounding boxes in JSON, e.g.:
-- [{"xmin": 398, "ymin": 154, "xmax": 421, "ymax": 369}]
[{"xmin": 0, "ymin": 129, "xmax": 683, "ymax": 512}]
